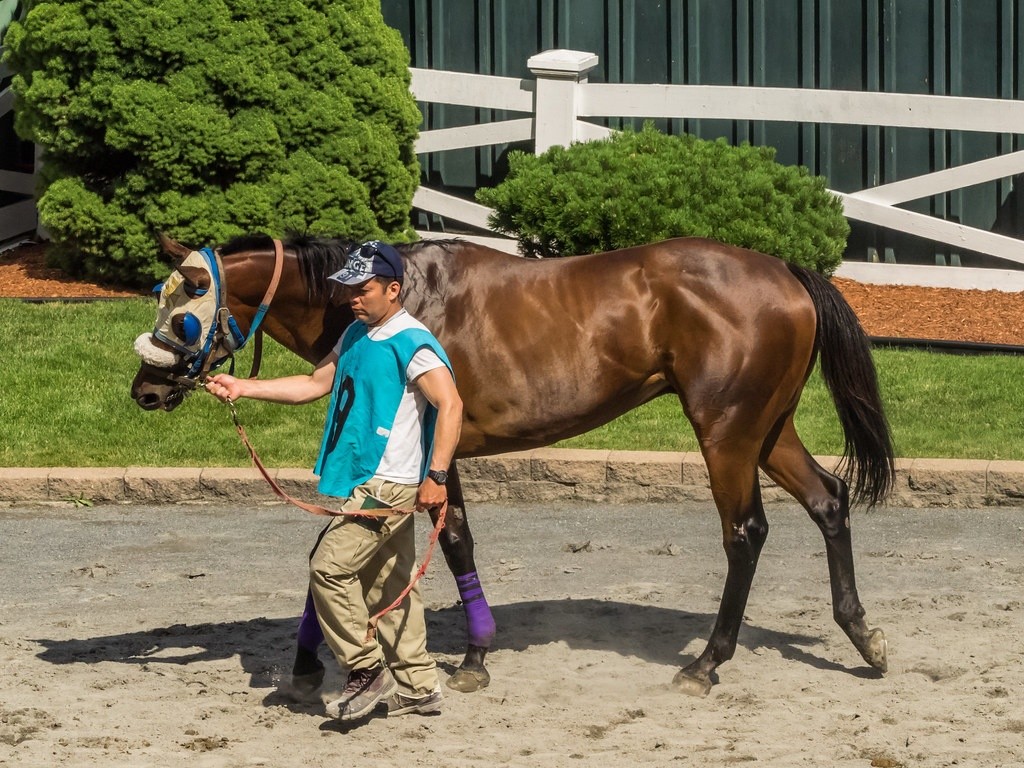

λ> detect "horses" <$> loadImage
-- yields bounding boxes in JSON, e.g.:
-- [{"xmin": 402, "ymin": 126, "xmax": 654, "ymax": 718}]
[{"xmin": 125, "ymin": 222, "xmax": 898, "ymax": 699}]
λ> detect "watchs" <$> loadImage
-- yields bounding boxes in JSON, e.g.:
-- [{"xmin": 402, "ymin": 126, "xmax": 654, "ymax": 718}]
[{"xmin": 428, "ymin": 469, "xmax": 448, "ymax": 485}]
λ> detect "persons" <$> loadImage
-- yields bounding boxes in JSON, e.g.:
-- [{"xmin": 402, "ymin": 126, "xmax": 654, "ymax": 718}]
[{"xmin": 211, "ymin": 240, "xmax": 465, "ymax": 720}]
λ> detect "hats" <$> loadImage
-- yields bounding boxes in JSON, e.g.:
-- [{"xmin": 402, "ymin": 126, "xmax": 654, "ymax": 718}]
[{"xmin": 327, "ymin": 240, "xmax": 404, "ymax": 286}]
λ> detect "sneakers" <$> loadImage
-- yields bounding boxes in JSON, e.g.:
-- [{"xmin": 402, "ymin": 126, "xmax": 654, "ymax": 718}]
[
  {"xmin": 374, "ymin": 692, "xmax": 446, "ymax": 716},
  {"xmin": 325, "ymin": 660, "xmax": 399, "ymax": 721}
]
